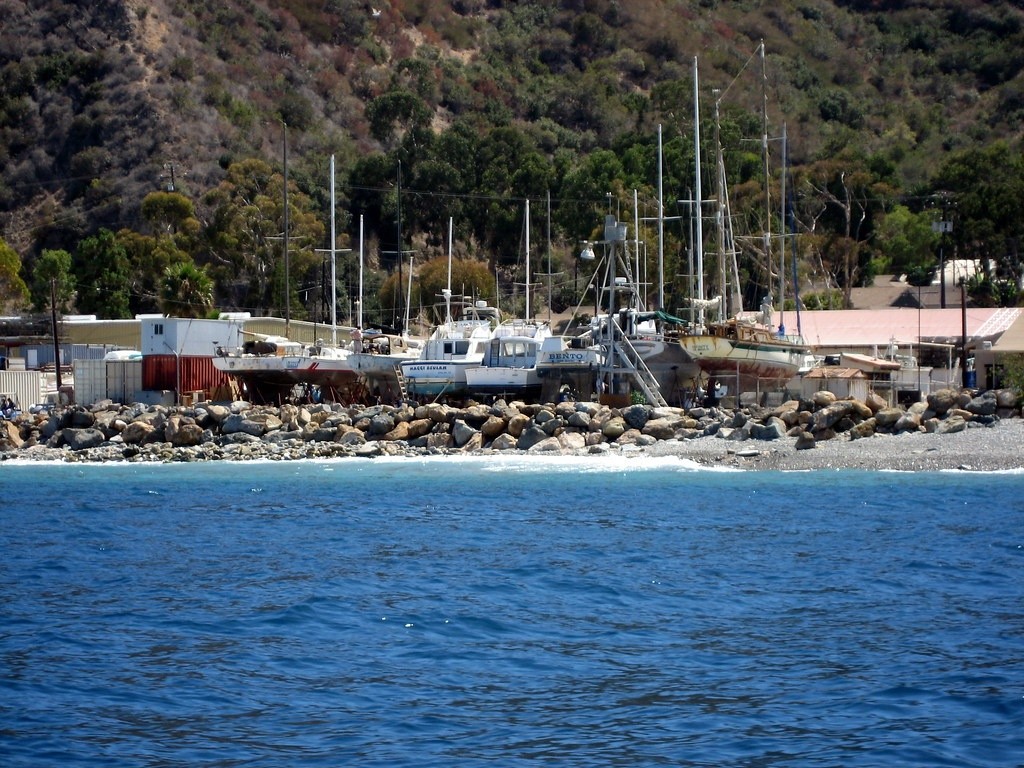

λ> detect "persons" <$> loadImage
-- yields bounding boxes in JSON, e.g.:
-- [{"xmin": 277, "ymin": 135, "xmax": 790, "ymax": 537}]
[
  {"xmin": 0, "ymin": 398, "xmax": 16, "ymax": 417},
  {"xmin": 395, "ymin": 315, "xmax": 403, "ymax": 336},
  {"xmin": 352, "ymin": 326, "xmax": 362, "ymax": 354}
]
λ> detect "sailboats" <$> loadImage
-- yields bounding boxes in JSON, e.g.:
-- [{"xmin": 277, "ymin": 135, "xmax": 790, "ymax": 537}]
[{"xmin": 211, "ymin": 37, "xmax": 816, "ymax": 405}]
